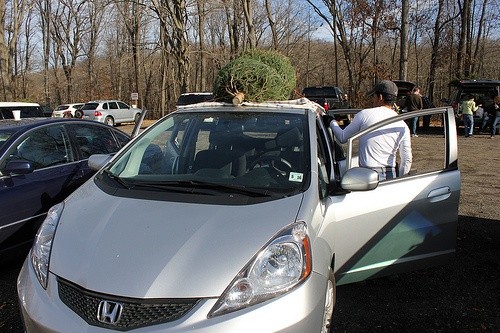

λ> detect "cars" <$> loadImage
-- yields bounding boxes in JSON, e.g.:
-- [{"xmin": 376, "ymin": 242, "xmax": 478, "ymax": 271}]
[
  {"xmin": 16, "ymin": 99, "xmax": 461, "ymax": 333},
  {"xmin": 80, "ymin": 99, "xmax": 142, "ymax": 127},
  {"xmin": 51, "ymin": 103, "xmax": 85, "ymax": 118},
  {"xmin": 0, "ymin": 117, "xmax": 169, "ymax": 254},
  {"xmin": 0, "ymin": 101, "xmax": 45, "ymax": 121}
]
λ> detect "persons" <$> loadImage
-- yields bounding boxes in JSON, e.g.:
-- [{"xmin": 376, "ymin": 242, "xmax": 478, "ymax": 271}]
[
  {"xmin": 324, "ymin": 81, "xmax": 412, "ymax": 181},
  {"xmin": 405, "ymin": 87, "xmax": 500, "ymax": 140}
]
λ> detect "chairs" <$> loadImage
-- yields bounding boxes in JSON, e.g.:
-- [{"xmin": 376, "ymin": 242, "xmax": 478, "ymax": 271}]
[
  {"xmin": 21, "ymin": 134, "xmax": 65, "ymax": 168},
  {"xmin": 192, "ymin": 126, "xmax": 246, "ymax": 177},
  {"xmin": 260, "ymin": 126, "xmax": 303, "ymax": 169}
]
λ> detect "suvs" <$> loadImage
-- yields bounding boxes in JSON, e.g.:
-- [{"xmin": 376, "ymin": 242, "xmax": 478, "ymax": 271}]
[
  {"xmin": 446, "ymin": 78, "xmax": 500, "ymax": 136},
  {"xmin": 173, "ymin": 92, "xmax": 215, "ymax": 128},
  {"xmin": 302, "ymin": 86, "xmax": 350, "ymax": 118},
  {"xmin": 393, "ymin": 79, "xmax": 420, "ymax": 131}
]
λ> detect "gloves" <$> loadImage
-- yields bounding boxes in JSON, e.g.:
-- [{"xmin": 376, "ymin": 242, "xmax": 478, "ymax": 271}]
[{"xmin": 322, "ymin": 115, "xmax": 334, "ymax": 128}]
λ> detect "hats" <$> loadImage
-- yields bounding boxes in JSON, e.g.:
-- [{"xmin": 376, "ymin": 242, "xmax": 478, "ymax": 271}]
[{"xmin": 366, "ymin": 80, "xmax": 398, "ymax": 96}]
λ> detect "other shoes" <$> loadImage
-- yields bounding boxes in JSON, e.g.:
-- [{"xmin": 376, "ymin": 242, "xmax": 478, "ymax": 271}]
[
  {"xmin": 388, "ymin": 275, "xmax": 399, "ymax": 281},
  {"xmin": 412, "ymin": 134, "xmax": 418, "ymax": 137},
  {"xmin": 469, "ymin": 135, "xmax": 473, "ymax": 138},
  {"xmin": 465, "ymin": 136, "xmax": 469, "ymax": 138},
  {"xmin": 374, "ymin": 279, "xmax": 380, "ymax": 286},
  {"xmin": 490, "ymin": 135, "xmax": 494, "ymax": 138}
]
